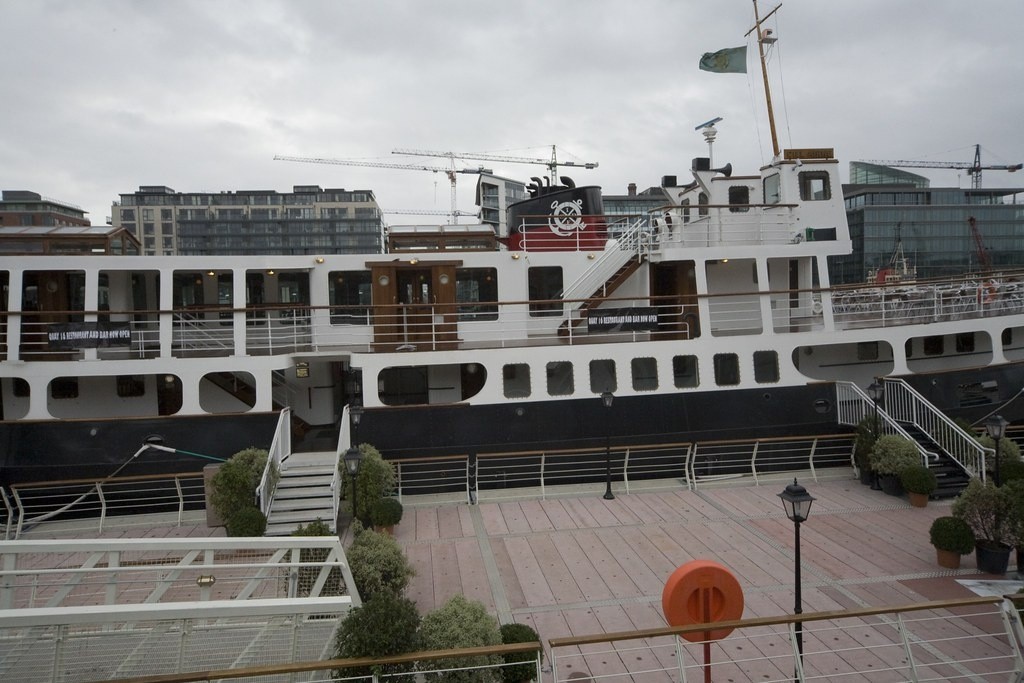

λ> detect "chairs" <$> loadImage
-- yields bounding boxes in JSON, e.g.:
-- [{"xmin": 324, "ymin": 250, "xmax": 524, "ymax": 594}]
[{"xmin": 829, "ymin": 285, "xmax": 1023, "ymax": 323}]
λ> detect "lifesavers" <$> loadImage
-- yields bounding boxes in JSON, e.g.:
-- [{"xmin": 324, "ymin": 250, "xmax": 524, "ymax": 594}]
[{"xmin": 976, "ymin": 282, "xmax": 995, "ymax": 305}]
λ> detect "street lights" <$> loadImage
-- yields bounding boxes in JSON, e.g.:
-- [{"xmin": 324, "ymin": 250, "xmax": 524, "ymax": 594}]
[
  {"xmin": 865, "ymin": 376, "xmax": 885, "ymax": 491},
  {"xmin": 982, "ymin": 412, "xmax": 1012, "ymax": 528},
  {"xmin": 775, "ymin": 475, "xmax": 816, "ymax": 682},
  {"xmin": 342, "ymin": 446, "xmax": 360, "ymax": 522},
  {"xmin": 598, "ymin": 387, "xmax": 616, "ymax": 500},
  {"xmin": 349, "ymin": 404, "xmax": 364, "ymax": 449}
]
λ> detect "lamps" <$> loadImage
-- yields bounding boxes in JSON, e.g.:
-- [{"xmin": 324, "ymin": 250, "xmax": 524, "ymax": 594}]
[
  {"xmin": 206, "ymin": 270, "xmax": 215, "ymax": 276},
  {"xmin": 587, "ymin": 253, "xmax": 595, "ymax": 259},
  {"xmin": 266, "ymin": 270, "xmax": 274, "ymax": 275},
  {"xmin": 409, "ymin": 259, "xmax": 418, "ymax": 264},
  {"xmin": 316, "ymin": 257, "xmax": 324, "ymax": 263},
  {"xmin": 511, "ymin": 254, "xmax": 520, "ymax": 259}
]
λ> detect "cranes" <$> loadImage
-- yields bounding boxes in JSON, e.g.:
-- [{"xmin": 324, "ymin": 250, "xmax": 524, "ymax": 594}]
[
  {"xmin": 274, "ymin": 155, "xmax": 493, "ymax": 226},
  {"xmin": 388, "ymin": 150, "xmax": 598, "ymax": 188},
  {"xmin": 860, "ymin": 143, "xmax": 1023, "ymax": 190}
]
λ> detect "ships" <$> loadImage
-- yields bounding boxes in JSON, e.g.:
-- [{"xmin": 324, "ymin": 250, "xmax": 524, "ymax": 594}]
[{"xmin": 0, "ymin": 0, "xmax": 1023, "ymax": 519}]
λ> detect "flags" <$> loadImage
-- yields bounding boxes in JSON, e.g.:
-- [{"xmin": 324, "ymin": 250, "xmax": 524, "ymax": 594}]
[{"xmin": 699, "ymin": 45, "xmax": 747, "ymax": 74}]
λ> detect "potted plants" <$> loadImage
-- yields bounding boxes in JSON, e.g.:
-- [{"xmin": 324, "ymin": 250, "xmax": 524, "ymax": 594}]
[
  {"xmin": 366, "ymin": 497, "xmax": 402, "ymax": 535},
  {"xmin": 929, "ymin": 417, "xmax": 1024, "ymax": 577},
  {"xmin": 227, "ymin": 506, "xmax": 267, "ymax": 554},
  {"xmin": 853, "ymin": 414, "xmax": 938, "ymax": 508}
]
